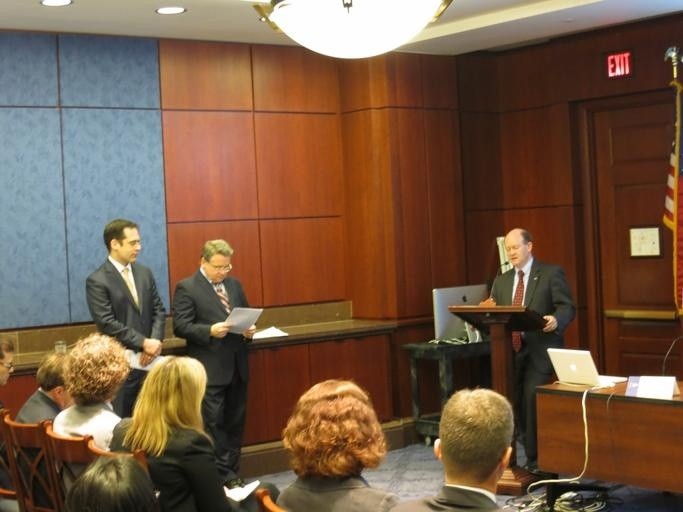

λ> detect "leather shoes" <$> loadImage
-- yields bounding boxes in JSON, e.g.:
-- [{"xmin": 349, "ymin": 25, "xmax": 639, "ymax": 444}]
[{"xmin": 227, "ymin": 478, "xmax": 242, "ymax": 489}]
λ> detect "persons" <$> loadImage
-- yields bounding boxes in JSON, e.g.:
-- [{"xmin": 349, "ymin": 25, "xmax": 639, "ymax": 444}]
[
  {"xmin": 0, "ymin": 331, "xmax": 19, "ymax": 488},
  {"xmin": 108, "ymin": 353, "xmax": 280, "ymax": 512},
  {"xmin": 385, "ymin": 385, "xmax": 516, "ymax": 511},
  {"xmin": 84, "ymin": 218, "xmax": 166, "ymax": 417},
  {"xmin": 53, "ymin": 332, "xmax": 134, "ymax": 496},
  {"xmin": 171, "ymin": 238, "xmax": 256, "ymax": 489},
  {"xmin": 272, "ymin": 379, "xmax": 405, "ymax": 511},
  {"xmin": 14, "ymin": 347, "xmax": 78, "ymax": 508},
  {"xmin": 61, "ymin": 452, "xmax": 160, "ymax": 512},
  {"xmin": 486, "ymin": 227, "xmax": 575, "ymax": 471}
]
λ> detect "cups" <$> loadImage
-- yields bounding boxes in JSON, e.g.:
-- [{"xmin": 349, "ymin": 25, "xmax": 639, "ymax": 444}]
[
  {"xmin": 465, "ymin": 320, "xmax": 481, "ymax": 343},
  {"xmin": 53, "ymin": 340, "xmax": 65, "ymax": 354}
]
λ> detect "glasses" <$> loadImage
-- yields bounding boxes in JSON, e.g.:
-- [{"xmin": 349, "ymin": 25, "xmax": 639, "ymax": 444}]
[
  {"xmin": 0, "ymin": 362, "xmax": 12, "ymax": 369},
  {"xmin": 208, "ymin": 262, "xmax": 233, "ymax": 272}
]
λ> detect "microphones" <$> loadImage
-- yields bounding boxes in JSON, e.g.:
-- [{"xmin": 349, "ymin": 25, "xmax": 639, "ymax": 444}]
[{"xmin": 479, "ymin": 262, "xmax": 509, "ymax": 306}]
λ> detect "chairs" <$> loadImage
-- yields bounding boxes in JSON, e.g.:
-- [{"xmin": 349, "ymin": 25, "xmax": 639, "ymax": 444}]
[
  {"xmin": 0, "ymin": 409, "xmax": 150, "ymax": 511},
  {"xmin": 255, "ymin": 488, "xmax": 288, "ymax": 512}
]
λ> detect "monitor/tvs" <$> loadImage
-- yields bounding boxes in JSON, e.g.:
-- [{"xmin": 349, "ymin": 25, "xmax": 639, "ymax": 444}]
[{"xmin": 432, "ymin": 284, "xmax": 488, "ymax": 340}]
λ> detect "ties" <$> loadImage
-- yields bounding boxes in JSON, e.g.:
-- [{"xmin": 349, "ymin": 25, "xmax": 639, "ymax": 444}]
[
  {"xmin": 119, "ymin": 268, "xmax": 138, "ymax": 306},
  {"xmin": 510, "ymin": 271, "xmax": 524, "ymax": 353},
  {"xmin": 215, "ymin": 285, "xmax": 231, "ymax": 316}
]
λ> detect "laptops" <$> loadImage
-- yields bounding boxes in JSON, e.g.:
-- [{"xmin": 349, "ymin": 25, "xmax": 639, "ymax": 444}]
[{"xmin": 547, "ymin": 348, "xmax": 628, "ymax": 386}]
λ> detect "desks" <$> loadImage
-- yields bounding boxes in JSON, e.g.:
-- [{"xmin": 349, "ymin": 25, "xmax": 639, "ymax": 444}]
[{"xmin": 534, "ymin": 380, "xmax": 683, "ymax": 511}]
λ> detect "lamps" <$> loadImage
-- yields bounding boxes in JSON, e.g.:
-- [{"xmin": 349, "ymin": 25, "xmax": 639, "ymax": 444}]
[{"xmin": 251, "ymin": 1, "xmax": 452, "ymax": 61}]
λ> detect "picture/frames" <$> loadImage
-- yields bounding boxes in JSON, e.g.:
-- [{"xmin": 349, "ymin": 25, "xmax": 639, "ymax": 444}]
[{"xmin": 629, "ymin": 223, "xmax": 664, "ymax": 259}]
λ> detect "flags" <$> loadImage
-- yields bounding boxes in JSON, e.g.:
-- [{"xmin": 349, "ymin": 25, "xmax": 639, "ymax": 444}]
[{"xmin": 659, "ymin": 78, "xmax": 683, "ymax": 317}]
